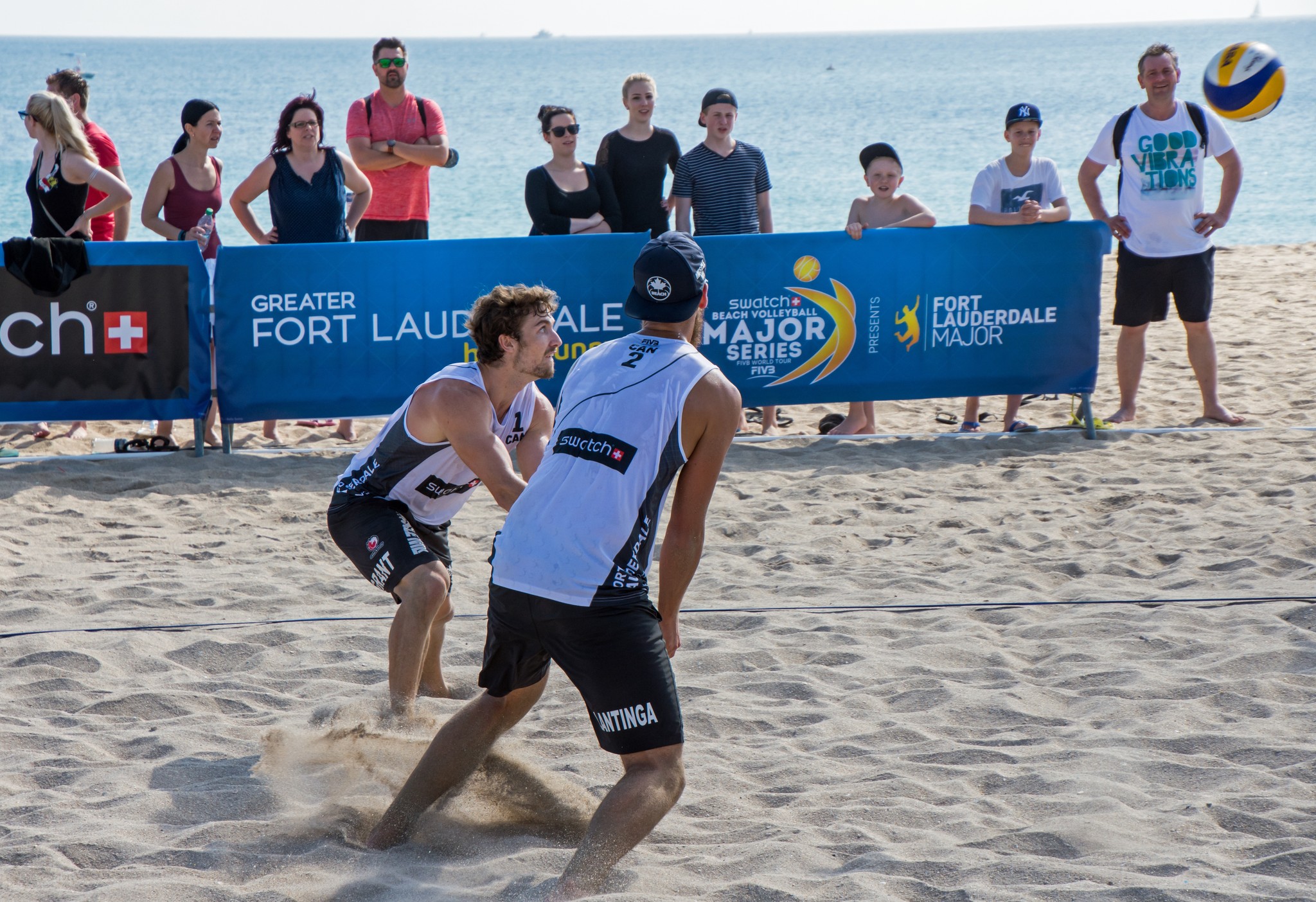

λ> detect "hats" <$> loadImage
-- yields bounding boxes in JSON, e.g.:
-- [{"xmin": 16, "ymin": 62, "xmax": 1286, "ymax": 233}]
[
  {"xmin": 1005, "ymin": 103, "xmax": 1043, "ymax": 129},
  {"xmin": 860, "ymin": 142, "xmax": 903, "ymax": 174},
  {"xmin": 698, "ymin": 88, "xmax": 738, "ymax": 128},
  {"xmin": 623, "ymin": 231, "xmax": 706, "ymax": 322}
]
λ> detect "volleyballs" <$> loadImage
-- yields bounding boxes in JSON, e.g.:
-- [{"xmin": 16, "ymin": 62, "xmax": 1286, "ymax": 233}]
[{"xmin": 1204, "ymin": 41, "xmax": 1286, "ymax": 122}]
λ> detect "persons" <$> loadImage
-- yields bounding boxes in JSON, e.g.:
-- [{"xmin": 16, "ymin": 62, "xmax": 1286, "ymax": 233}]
[
  {"xmin": 229, "ymin": 86, "xmax": 372, "ymax": 447},
  {"xmin": 1077, "ymin": 40, "xmax": 1247, "ymax": 425},
  {"xmin": 523, "ymin": 103, "xmax": 618, "ymax": 236},
  {"xmin": 953, "ymin": 102, "xmax": 1072, "ymax": 435},
  {"xmin": 595, "ymin": 71, "xmax": 683, "ymax": 242},
  {"xmin": 140, "ymin": 99, "xmax": 225, "ymax": 448},
  {"xmin": 822, "ymin": 142, "xmax": 937, "ymax": 441},
  {"xmin": 365, "ymin": 230, "xmax": 744, "ymax": 902},
  {"xmin": 327, "ymin": 282, "xmax": 561, "ymax": 718},
  {"xmin": 17, "ymin": 68, "xmax": 133, "ymax": 440},
  {"xmin": 670, "ymin": 89, "xmax": 778, "ymax": 439},
  {"xmin": 355, "ymin": 36, "xmax": 449, "ymax": 242}
]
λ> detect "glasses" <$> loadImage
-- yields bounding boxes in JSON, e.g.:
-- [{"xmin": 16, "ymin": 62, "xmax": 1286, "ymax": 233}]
[
  {"xmin": 548, "ymin": 123, "xmax": 580, "ymax": 138},
  {"xmin": 289, "ymin": 119, "xmax": 320, "ymax": 129},
  {"xmin": 18, "ymin": 110, "xmax": 39, "ymax": 122},
  {"xmin": 375, "ymin": 57, "xmax": 405, "ymax": 69}
]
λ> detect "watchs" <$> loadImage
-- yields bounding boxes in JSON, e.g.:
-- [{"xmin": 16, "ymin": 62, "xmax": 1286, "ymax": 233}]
[{"xmin": 387, "ymin": 138, "xmax": 394, "ymax": 154}]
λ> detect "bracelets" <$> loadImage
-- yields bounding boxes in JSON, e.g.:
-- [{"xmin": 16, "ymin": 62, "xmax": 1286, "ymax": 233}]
[{"xmin": 177, "ymin": 229, "xmax": 186, "ymax": 240}]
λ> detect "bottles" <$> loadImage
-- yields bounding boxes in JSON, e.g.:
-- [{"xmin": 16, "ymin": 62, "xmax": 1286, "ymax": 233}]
[
  {"xmin": 91, "ymin": 438, "xmax": 129, "ymax": 454},
  {"xmin": 195, "ymin": 207, "xmax": 214, "ymax": 253}
]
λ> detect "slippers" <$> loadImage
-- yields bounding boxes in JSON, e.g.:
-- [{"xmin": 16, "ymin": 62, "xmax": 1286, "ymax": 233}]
[
  {"xmin": 0, "ymin": 447, "xmax": 19, "ymax": 457},
  {"xmin": 960, "ymin": 422, "xmax": 981, "ymax": 432},
  {"xmin": 935, "ymin": 411, "xmax": 958, "ymax": 424},
  {"xmin": 1009, "ymin": 421, "xmax": 1038, "ymax": 432},
  {"xmin": 297, "ymin": 418, "xmax": 336, "ymax": 427},
  {"xmin": 1021, "ymin": 394, "xmax": 1060, "ymax": 405},
  {"xmin": 122, "ymin": 434, "xmax": 180, "ymax": 452},
  {"xmin": 744, "ymin": 406, "xmax": 792, "ymax": 426},
  {"xmin": 979, "ymin": 412, "xmax": 1003, "ymax": 422}
]
